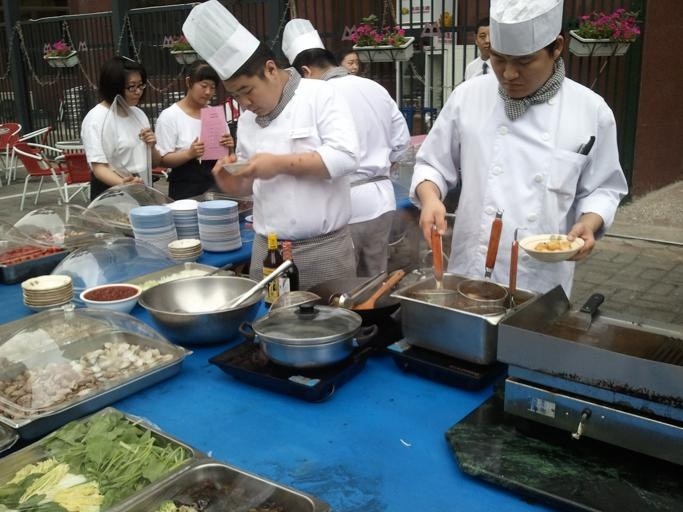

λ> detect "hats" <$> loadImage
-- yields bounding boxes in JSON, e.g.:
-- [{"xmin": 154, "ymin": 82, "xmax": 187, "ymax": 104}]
[
  {"xmin": 281, "ymin": 18, "xmax": 325, "ymax": 65},
  {"xmin": 489, "ymin": 0, "xmax": 564, "ymax": 56},
  {"xmin": 180, "ymin": 1, "xmax": 260, "ymax": 80}
]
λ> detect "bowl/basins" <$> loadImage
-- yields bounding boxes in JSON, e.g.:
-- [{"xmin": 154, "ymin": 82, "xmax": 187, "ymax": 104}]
[
  {"xmin": 138, "ymin": 276, "xmax": 263, "ymax": 346},
  {"xmin": 518, "ymin": 235, "xmax": 586, "ymax": 264},
  {"xmin": 79, "ymin": 284, "xmax": 141, "ymax": 317}
]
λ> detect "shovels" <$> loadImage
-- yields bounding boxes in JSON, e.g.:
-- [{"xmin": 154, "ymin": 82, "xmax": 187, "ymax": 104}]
[
  {"xmin": 555, "ymin": 293, "xmax": 605, "ymax": 332},
  {"xmin": 353, "ymin": 270, "xmax": 405, "ymax": 310}
]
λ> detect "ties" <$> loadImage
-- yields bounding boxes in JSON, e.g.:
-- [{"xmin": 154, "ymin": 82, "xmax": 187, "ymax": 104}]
[{"xmin": 482, "ymin": 62, "xmax": 488, "ymax": 73}]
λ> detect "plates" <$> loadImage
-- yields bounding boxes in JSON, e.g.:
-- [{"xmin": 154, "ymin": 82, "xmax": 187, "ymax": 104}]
[
  {"xmin": 22, "ymin": 275, "xmax": 75, "ymax": 310},
  {"xmin": 221, "ymin": 163, "xmax": 251, "ymax": 175},
  {"xmin": 128, "ymin": 198, "xmax": 243, "ymax": 263}
]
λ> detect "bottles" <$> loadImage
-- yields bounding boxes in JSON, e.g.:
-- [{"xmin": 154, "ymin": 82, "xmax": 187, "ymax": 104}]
[{"xmin": 262, "ymin": 233, "xmax": 300, "ymax": 308}]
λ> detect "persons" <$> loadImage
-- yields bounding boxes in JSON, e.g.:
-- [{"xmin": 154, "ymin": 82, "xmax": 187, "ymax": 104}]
[
  {"xmin": 280, "ymin": 17, "xmax": 411, "ymax": 276},
  {"xmin": 154, "ymin": 60, "xmax": 234, "ymax": 202},
  {"xmin": 335, "ymin": 48, "xmax": 360, "ymax": 75},
  {"xmin": 80, "ymin": 57, "xmax": 157, "ymax": 203},
  {"xmin": 182, "ymin": 0, "xmax": 359, "ymax": 292},
  {"xmin": 409, "ymin": 0, "xmax": 629, "ymax": 300},
  {"xmin": 464, "ymin": 17, "xmax": 496, "ymax": 81}
]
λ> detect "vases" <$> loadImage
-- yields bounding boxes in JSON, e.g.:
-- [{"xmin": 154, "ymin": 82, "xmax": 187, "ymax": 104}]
[
  {"xmin": 569, "ymin": 30, "xmax": 636, "ymax": 57},
  {"xmin": 353, "ymin": 37, "xmax": 415, "ymax": 63},
  {"xmin": 43, "ymin": 51, "xmax": 80, "ymax": 67},
  {"xmin": 170, "ymin": 49, "xmax": 204, "ymax": 64}
]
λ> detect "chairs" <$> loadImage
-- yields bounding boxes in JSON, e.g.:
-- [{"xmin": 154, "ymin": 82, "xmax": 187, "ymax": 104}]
[{"xmin": 0, "ymin": 122, "xmax": 171, "ymax": 225}]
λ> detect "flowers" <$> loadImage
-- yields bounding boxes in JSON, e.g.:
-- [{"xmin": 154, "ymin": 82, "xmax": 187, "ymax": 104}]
[
  {"xmin": 351, "ymin": 13, "xmax": 406, "ymax": 48},
  {"xmin": 169, "ymin": 35, "xmax": 194, "ymax": 51},
  {"xmin": 575, "ymin": 9, "xmax": 643, "ymax": 47},
  {"xmin": 47, "ymin": 40, "xmax": 71, "ymax": 57}
]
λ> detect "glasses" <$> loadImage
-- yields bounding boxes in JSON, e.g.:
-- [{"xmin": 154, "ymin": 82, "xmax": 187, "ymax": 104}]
[{"xmin": 119, "ymin": 85, "xmax": 146, "ymax": 91}]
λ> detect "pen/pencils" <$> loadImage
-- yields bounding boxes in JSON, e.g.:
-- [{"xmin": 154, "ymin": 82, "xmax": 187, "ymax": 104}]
[{"xmin": 199, "ymin": 157, "xmax": 202, "ymax": 166}]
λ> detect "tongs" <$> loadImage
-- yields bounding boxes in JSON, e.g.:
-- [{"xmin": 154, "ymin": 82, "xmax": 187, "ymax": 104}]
[{"xmin": 330, "ymin": 269, "xmax": 388, "ymax": 306}]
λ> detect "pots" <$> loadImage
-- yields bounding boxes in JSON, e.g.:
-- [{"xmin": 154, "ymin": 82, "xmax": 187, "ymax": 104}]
[
  {"xmin": 238, "ymin": 304, "xmax": 380, "ymax": 368},
  {"xmin": 306, "ymin": 262, "xmax": 413, "ymax": 318}
]
